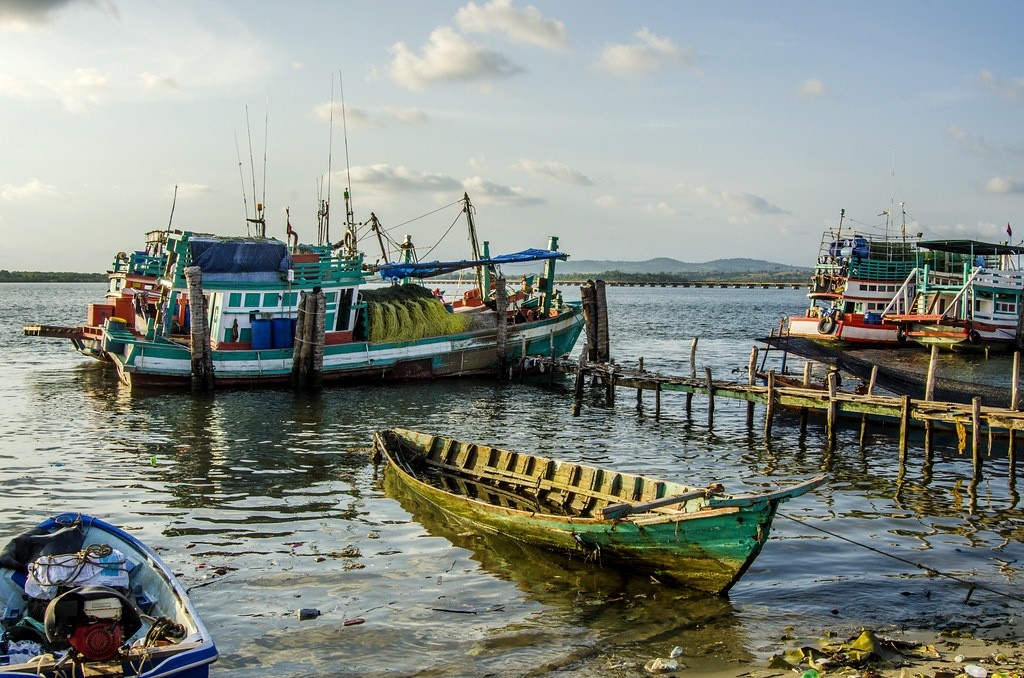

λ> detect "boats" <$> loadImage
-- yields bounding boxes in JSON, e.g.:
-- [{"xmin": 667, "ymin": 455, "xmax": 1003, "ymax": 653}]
[
  {"xmin": 776, "ymin": 169, "xmax": 936, "ymax": 345},
  {"xmin": 371, "ymin": 426, "xmax": 829, "ymax": 595},
  {"xmin": 0, "ymin": 512, "xmax": 221, "ymax": 678},
  {"xmin": 882, "ymin": 237, "xmax": 1024, "ymax": 356},
  {"xmin": 23, "ymin": 70, "xmax": 587, "ymax": 388}
]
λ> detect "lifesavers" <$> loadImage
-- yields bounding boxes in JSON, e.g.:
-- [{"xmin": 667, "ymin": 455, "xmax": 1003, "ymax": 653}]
[
  {"xmin": 344, "ymin": 229, "xmax": 355, "ymax": 251},
  {"xmin": 897, "ymin": 326, "xmax": 906, "ymax": 343},
  {"xmin": 818, "ymin": 316, "xmax": 836, "ymax": 335},
  {"xmin": 969, "ymin": 331, "xmax": 982, "ymax": 345}
]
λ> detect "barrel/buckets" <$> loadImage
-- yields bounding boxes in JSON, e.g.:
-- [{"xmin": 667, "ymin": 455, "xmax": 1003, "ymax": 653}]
[
  {"xmin": 103, "ymin": 317, "xmax": 127, "ymax": 353},
  {"xmin": 272, "ymin": 317, "xmax": 294, "ymax": 349},
  {"xmin": 292, "ymin": 318, "xmax": 297, "ymax": 331},
  {"xmin": 251, "ymin": 319, "xmax": 272, "ymax": 350}
]
